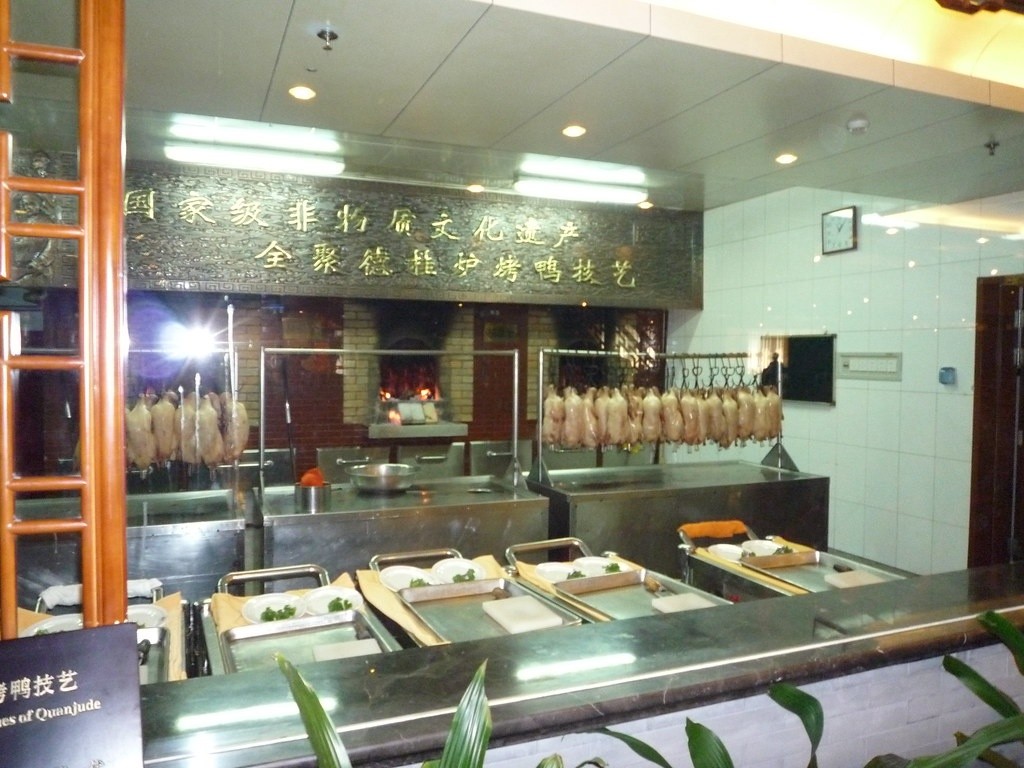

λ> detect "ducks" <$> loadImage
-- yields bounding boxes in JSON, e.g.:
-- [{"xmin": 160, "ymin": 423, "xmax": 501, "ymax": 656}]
[
  {"xmin": 125, "ymin": 390, "xmax": 249, "ymax": 478},
  {"xmin": 543, "ymin": 383, "xmax": 785, "ymax": 445}
]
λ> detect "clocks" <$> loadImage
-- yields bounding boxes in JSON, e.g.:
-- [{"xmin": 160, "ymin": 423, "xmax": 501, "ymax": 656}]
[{"xmin": 820, "ymin": 205, "xmax": 857, "ymax": 255}]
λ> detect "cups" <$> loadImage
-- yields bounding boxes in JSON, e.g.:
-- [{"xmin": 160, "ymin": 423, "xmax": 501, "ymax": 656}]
[{"xmin": 295, "ymin": 483, "xmax": 331, "ymax": 514}]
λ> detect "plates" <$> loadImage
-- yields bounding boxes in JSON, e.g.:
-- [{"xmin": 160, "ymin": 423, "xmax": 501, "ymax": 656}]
[
  {"xmin": 127, "ymin": 604, "xmax": 167, "ymax": 627},
  {"xmin": 302, "ymin": 586, "xmax": 363, "ymax": 616},
  {"xmin": 573, "ymin": 557, "xmax": 632, "ymax": 578},
  {"xmin": 379, "ymin": 565, "xmax": 440, "ymax": 592},
  {"xmin": 242, "ymin": 593, "xmax": 306, "ymax": 624},
  {"xmin": 537, "ymin": 563, "xmax": 576, "ymax": 584},
  {"xmin": 742, "ymin": 540, "xmax": 782, "ymax": 557},
  {"xmin": 19, "ymin": 613, "xmax": 84, "ymax": 638},
  {"xmin": 432, "ymin": 558, "xmax": 486, "ymax": 584},
  {"xmin": 708, "ymin": 544, "xmax": 743, "ymax": 562}
]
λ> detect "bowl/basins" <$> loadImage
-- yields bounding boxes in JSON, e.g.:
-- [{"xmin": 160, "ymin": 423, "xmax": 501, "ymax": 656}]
[{"xmin": 346, "ymin": 463, "xmax": 420, "ymax": 490}]
